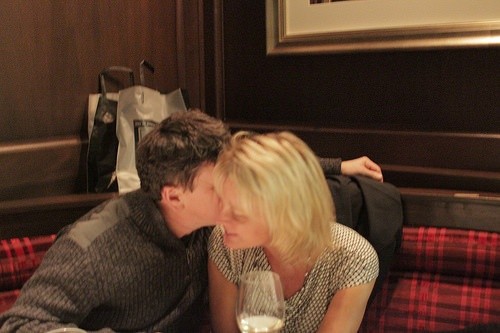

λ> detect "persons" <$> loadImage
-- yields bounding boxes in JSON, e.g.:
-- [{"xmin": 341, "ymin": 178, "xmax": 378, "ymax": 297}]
[
  {"xmin": 0, "ymin": 108, "xmax": 383, "ymax": 333},
  {"xmin": 208, "ymin": 131, "xmax": 379, "ymax": 332}
]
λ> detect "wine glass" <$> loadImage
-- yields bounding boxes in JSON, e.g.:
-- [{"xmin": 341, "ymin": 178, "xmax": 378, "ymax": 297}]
[{"xmin": 235, "ymin": 271, "xmax": 285, "ymax": 333}]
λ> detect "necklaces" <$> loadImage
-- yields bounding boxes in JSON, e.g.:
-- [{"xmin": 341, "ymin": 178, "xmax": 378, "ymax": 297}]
[{"xmin": 304, "ymin": 251, "xmax": 312, "ymax": 279}]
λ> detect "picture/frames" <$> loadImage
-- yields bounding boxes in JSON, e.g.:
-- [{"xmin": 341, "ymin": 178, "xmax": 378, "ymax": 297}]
[{"xmin": 265, "ymin": 0, "xmax": 500, "ymax": 55}]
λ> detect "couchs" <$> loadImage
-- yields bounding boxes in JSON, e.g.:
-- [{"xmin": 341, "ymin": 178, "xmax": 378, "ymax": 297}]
[{"xmin": 0, "ymin": 189, "xmax": 500, "ymax": 333}]
